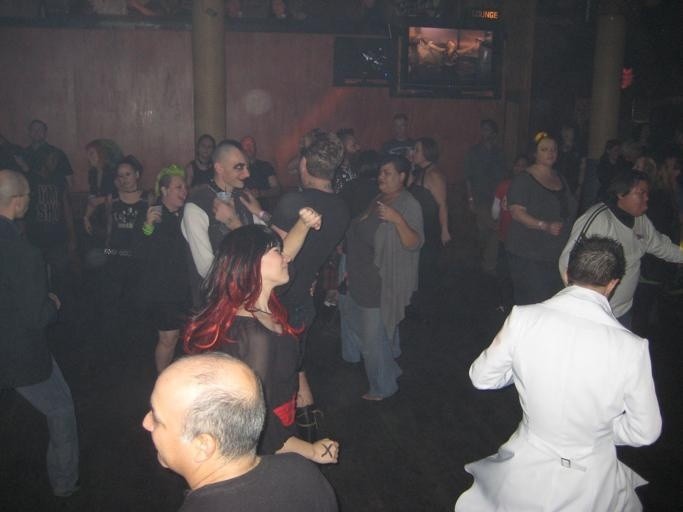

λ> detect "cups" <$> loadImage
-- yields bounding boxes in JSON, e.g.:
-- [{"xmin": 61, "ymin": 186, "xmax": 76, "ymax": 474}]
[
  {"xmin": 152, "ymin": 205, "xmax": 163, "ymax": 224},
  {"xmin": 216, "ymin": 191, "xmax": 231, "ymax": 204}
]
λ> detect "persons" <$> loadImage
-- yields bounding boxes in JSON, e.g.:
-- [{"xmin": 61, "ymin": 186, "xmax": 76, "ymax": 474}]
[
  {"xmin": 0, "ymin": 1, "xmax": 295, "ymax": 21},
  {"xmin": 451, "ymin": 233, "xmax": 663, "ymax": 510},
  {"xmin": 167, "ymin": 225, "xmax": 340, "ymax": 494},
  {"xmin": 1, "ymin": 113, "xmax": 683, "ymax": 445},
  {"xmin": 418, "ymin": 36, "xmax": 482, "ymax": 83},
  {"xmin": 0, "ymin": 170, "xmax": 99, "ymax": 511},
  {"xmin": 141, "ymin": 348, "xmax": 343, "ymax": 511}
]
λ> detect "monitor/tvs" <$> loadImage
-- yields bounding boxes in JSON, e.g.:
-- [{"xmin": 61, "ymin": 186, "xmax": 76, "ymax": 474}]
[
  {"xmin": 333, "ymin": 35, "xmax": 390, "ymax": 86},
  {"xmin": 388, "ymin": 16, "xmax": 502, "ymax": 98}
]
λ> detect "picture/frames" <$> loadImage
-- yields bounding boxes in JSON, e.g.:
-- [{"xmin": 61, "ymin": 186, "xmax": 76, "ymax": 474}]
[{"xmin": 388, "ymin": 21, "xmax": 509, "ymax": 103}]
